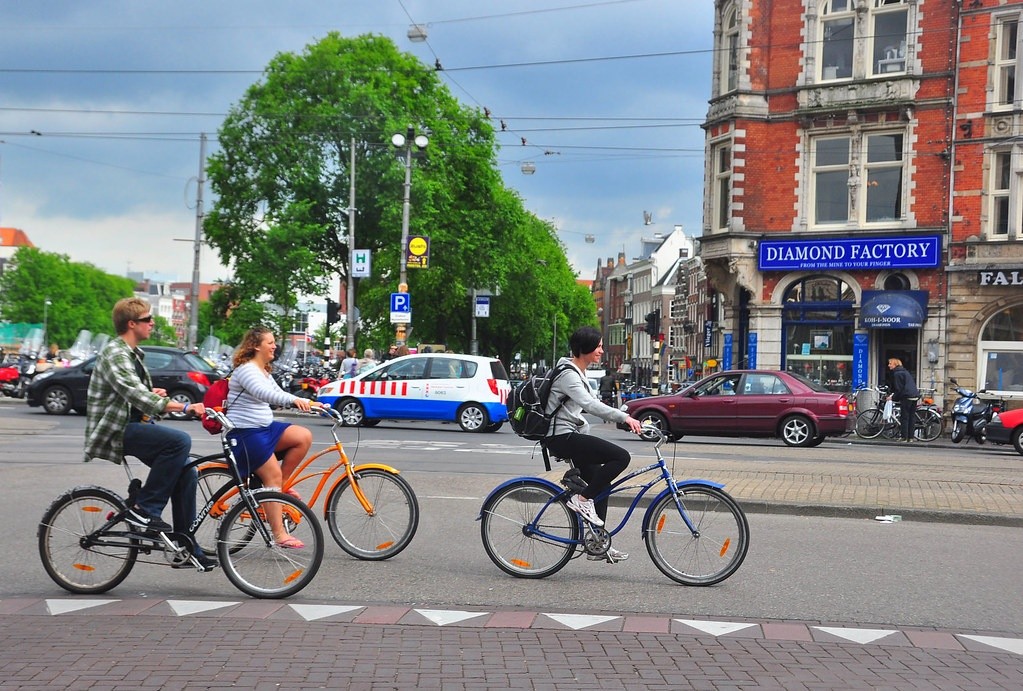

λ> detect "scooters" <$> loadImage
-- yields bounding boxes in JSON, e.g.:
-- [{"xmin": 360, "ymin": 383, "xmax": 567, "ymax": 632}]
[
  {"xmin": 0, "ymin": 326, "xmax": 113, "ymax": 399},
  {"xmin": 198, "ymin": 336, "xmax": 338, "ymax": 408},
  {"xmin": 950, "ymin": 377, "xmax": 1012, "ymax": 445}
]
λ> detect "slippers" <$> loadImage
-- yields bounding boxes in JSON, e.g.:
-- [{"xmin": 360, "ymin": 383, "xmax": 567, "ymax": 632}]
[
  {"xmin": 282, "ymin": 484, "xmax": 302, "ymax": 502},
  {"xmin": 274, "ymin": 533, "xmax": 304, "ymax": 550}
]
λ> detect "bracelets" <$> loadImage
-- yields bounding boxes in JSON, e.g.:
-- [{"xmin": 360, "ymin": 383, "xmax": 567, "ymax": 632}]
[
  {"xmin": 183, "ymin": 403, "xmax": 189, "ymax": 415},
  {"xmin": 308, "ymin": 401, "xmax": 314, "ymax": 406}
]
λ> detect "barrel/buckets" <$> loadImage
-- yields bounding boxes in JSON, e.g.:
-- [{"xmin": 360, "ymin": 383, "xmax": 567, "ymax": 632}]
[{"xmin": 856, "ymin": 390, "xmax": 880, "ymax": 436}]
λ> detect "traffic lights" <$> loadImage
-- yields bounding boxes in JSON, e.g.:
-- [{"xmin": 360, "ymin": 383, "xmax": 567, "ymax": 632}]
[
  {"xmin": 645, "ymin": 314, "xmax": 655, "ymax": 334},
  {"xmin": 328, "ymin": 302, "xmax": 341, "ymax": 322}
]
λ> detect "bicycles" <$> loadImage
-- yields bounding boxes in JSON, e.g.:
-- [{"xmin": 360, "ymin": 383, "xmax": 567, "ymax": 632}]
[
  {"xmin": 190, "ymin": 403, "xmax": 420, "ymax": 563},
  {"xmin": 601, "ymin": 391, "xmax": 617, "ymax": 424},
  {"xmin": 476, "ymin": 422, "xmax": 750, "ymax": 586},
  {"xmin": 36, "ymin": 408, "xmax": 326, "ymax": 599},
  {"xmin": 619, "ymin": 381, "xmax": 652, "ymax": 403},
  {"xmin": 836, "ymin": 384, "xmax": 943, "ymax": 442}
]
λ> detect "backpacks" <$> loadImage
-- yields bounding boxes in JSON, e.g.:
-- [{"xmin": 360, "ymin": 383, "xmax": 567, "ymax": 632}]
[
  {"xmin": 201, "ymin": 362, "xmax": 245, "ymax": 435},
  {"xmin": 506, "ymin": 364, "xmax": 581, "ymax": 441}
]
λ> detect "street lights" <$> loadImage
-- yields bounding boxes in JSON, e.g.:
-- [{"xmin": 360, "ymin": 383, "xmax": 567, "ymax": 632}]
[
  {"xmin": 43, "ymin": 294, "xmax": 52, "ymax": 340},
  {"xmin": 389, "ymin": 133, "xmax": 429, "ymax": 346}
]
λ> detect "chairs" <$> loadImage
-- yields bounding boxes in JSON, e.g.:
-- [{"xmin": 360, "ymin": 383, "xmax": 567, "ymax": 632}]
[{"xmin": 751, "ymin": 383, "xmax": 764, "ymax": 394}]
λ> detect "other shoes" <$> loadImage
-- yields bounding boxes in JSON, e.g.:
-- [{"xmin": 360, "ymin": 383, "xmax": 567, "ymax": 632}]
[
  {"xmin": 907, "ymin": 438, "xmax": 913, "ymax": 442},
  {"xmin": 896, "ymin": 437, "xmax": 906, "ymax": 442}
]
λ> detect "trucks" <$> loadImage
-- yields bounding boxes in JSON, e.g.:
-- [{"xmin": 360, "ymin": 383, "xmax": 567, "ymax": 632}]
[{"xmin": 585, "ymin": 370, "xmax": 606, "ymax": 399}]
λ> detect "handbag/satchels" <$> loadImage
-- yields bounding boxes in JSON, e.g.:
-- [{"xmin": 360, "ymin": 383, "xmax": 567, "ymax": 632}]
[{"xmin": 881, "ymin": 398, "xmax": 892, "ymax": 421}]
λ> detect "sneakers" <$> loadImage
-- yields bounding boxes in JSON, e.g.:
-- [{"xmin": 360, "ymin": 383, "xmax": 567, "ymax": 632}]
[
  {"xmin": 169, "ymin": 549, "xmax": 218, "ymax": 570},
  {"xmin": 565, "ymin": 493, "xmax": 605, "ymax": 526},
  {"xmin": 125, "ymin": 504, "xmax": 173, "ymax": 532},
  {"xmin": 586, "ymin": 544, "xmax": 629, "ymax": 561}
]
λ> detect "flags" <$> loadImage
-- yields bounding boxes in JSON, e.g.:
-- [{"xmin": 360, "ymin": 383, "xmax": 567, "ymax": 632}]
[{"xmin": 659, "ymin": 340, "xmax": 667, "ymax": 356}]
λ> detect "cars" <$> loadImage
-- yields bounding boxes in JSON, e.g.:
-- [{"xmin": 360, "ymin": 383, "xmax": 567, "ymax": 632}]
[
  {"xmin": 317, "ymin": 352, "xmax": 511, "ymax": 433},
  {"xmin": 983, "ymin": 408, "xmax": 1023, "ymax": 454},
  {"xmin": 616, "ymin": 368, "xmax": 857, "ymax": 447},
  {"xmin": 29, "ymin": 346, "xmax": 222, "ymax": 420}
]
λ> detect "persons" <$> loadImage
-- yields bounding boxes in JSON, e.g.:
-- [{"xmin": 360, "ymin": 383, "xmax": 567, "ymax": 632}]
[
  {"xmin": 0, "ymin": 342, "xmax": 69, "ymax": 400},
  {"xmin": 543, "ymin": 327, "xmax": 642, "ymax": 562},
  {"xmin": 330, "ymin": 344, "xmax": 457, "ymax": 423},
  {"xmin": 221, "ymin": 324, "xmax": 324, "ymax": 549},
  {"xmin": 887, "ymin": 358, "xmax": 920, "ymax": 442},
  {"xmin": 84, "ymin": 297, "xmax": 219, "ymax": 569},
  {"xmin": 597, "ymin": 368, "xmax": 619, "ymax": 407}
]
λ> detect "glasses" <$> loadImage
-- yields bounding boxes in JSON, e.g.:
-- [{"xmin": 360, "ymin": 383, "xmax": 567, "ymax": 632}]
[{"xmin": 132, "ymin": 315, "xmax": 153, "ymax": 322}]
